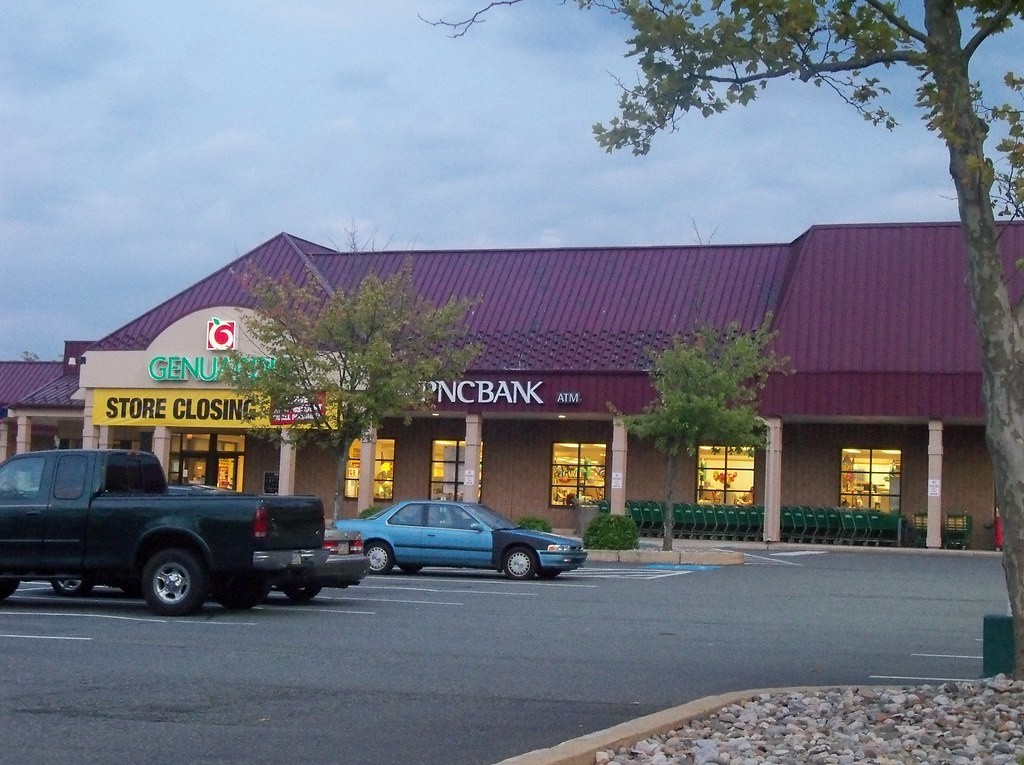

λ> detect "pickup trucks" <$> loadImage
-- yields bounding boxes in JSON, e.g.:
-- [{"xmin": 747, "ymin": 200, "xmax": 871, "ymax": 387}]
[{"xmin": 1, "ymin": 449, "xmax": 329, "ymax": 617}]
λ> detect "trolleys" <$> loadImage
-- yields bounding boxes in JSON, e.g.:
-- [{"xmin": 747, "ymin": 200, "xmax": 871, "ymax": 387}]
[
  {"xmin": 941, "ymin": 511, "xmax": 974, "ymax": 549},
  {"xmin": 594, "ymin": 499, "xmax": 899, "ymax": 546},
  {"xmin": 913, "ymin": 511, "xmax": 927, "ymax": 545}
]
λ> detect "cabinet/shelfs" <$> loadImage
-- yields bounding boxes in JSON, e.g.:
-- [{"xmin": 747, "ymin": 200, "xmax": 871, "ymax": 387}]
[{"xmin": 889, "ymin": 476, "xmax": 900, "ymax": 511}]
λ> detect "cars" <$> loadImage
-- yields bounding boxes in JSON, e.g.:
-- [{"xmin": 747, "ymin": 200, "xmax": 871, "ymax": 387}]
[
  {"xmin": 335, "ymin": 499, "xmax": 589, "ymax": 580},
  {"xmin": 50, "ymin": 483, "xmax": 371, "ymax": 601}
]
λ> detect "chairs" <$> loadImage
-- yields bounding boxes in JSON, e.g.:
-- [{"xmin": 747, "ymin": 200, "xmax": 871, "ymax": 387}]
[{"xmin": 445, "ymin": 508, "xmax": 460, "ymax": 528}]
